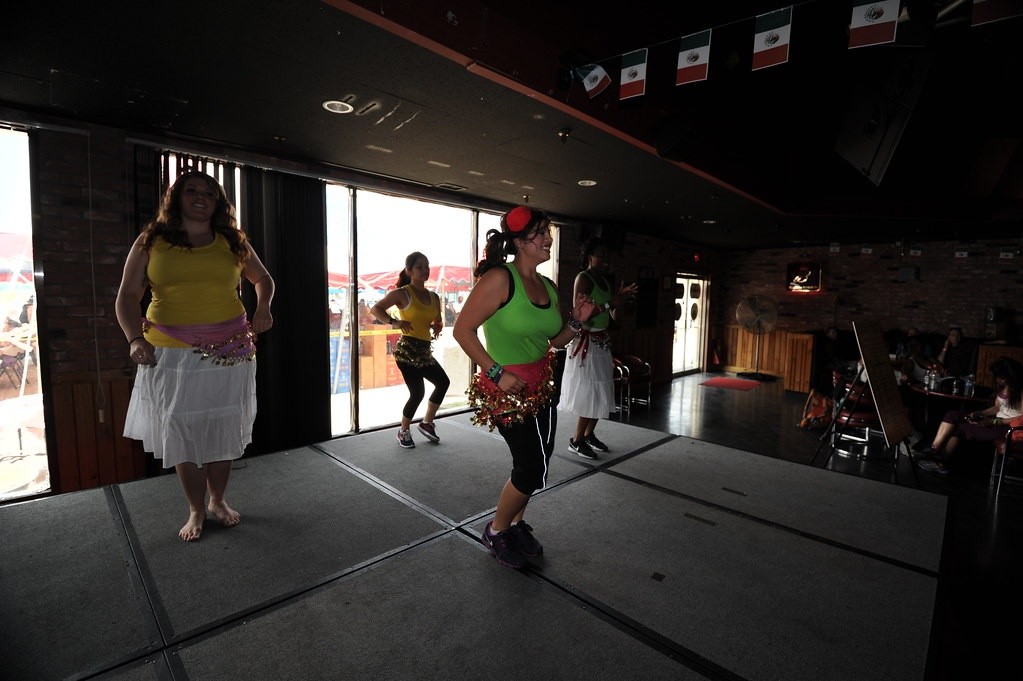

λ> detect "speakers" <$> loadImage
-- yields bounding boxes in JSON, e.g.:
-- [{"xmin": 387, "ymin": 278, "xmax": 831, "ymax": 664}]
[
  {"xmin": 652, "ymin": 116, "xmax": 702, "ymax": 160},
  {"xmin": 834, "ymin": 0, "xmax": 936, "ymax": 185}
]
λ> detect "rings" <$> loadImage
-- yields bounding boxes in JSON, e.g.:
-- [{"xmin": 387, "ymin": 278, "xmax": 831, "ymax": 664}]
[{"xmin": 138, "ymin": 355, "xmax": 144, "ymax": 359}]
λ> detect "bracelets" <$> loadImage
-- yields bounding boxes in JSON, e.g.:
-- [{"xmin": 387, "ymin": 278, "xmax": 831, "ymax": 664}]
[
  {"xmin": 597, "ymin": 305, "xmax": 603, "ymax": 313},
  {"xmin": 129, "ymin": 336, "xmax": 144, "ymax": 346},
  {"xmin": 604, "ymin": 302, "xmax": 610, "ymax": 309},
  {"xmin": 992, "ymin": 417, "xmax": 1003, "ymax": 425},
  {"xmin": 567, "ymin": 317, "xmax": 583, "ymax": 332},
  {"xmin": 389, "ymin": 318, "xmax": 395, "ymax": 324},
  {"xmin": 485, "ymin": 362, "xmax": 505, "ymax": 382},
  {"xmin": 942, "ymin": 348, "xmax": 947, "ymax": 351}
]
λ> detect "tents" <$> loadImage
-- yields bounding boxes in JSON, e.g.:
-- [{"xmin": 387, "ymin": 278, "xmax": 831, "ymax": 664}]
[
  {"xmin": 326, "ymin": 264, "xmax": 478, "ymax": 393},
  {"xmin": 0, "ymin": 232, "xmax": 41, "ymax": 397}
]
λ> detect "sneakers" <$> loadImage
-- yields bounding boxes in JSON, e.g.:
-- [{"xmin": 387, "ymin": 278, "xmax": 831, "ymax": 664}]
[
  {"xmin": 482, "ymin": 520, "xmax": 543, "ymax": 569},
  {"xmin": 583, "ymin": 432, "xmax": 608, "ymax": 451},
  {"xmin": 912, "ymin": 448, "xmax": 951, "ymax": 475},
  {"xmin": 568, "ymin": 438, "xmax": 598, "ymax": 460},
  {"xmin": 397, "ymin": 428, "xmax": 414, "ymax": 448},
  {"xmin": 417, "ymin": 421, "xmax": 440, "ymax": 442}
]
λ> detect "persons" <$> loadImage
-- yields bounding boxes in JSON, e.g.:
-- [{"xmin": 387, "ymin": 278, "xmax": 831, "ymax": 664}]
[
  {"xmin": 328, "ymin": 299, "xmax": 383, "ymax": 324},
  {"xmin": 557, "ymin": 238, "xmax": 638, "ymax": 458},
  {"xmin": 445, "ymin": 296, "xmax": 465, "ymax": 326},
  {"xmin": 18, "ymin": 295, "xmax": 33, "ymax": 324},
  {"xmin": 369, "ymin": 252, "xmax": 451, "ymax": 448},
  {"xmin": 453, "ymin": 206, "xmax": 595, "ymax": 569},
  {"xmin": 115, "ymin": 171, "xmax": 275, "ymax": 541},
  {"xmin": 812, "ymin": 327, "xmax": 1023, "ymax": 474}
]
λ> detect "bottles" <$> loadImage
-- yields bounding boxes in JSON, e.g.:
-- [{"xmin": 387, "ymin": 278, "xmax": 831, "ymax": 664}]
[
  {"xmin": 924, "ymin": 370, "xmax": 930, "ymax": 391},
  {"xmin": 953, "ymin": 380, "xmax": 961, "ymax": 394},
  {"xmin": 964, "ymin": 372, "xmax": 976, "ymax": 397},
  {"xmin": 930, "ymin": 364, "xmax": 940, "ymax": 391}
]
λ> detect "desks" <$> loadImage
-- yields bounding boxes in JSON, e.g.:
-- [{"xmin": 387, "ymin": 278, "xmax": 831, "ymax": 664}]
[{"xmin": 911, "ymin": 383, "xmax": 996, "ymax": 424}]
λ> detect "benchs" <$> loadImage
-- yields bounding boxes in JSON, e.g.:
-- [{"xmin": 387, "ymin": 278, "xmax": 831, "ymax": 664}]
[{"xmin": 785, "ymin": 323, "xmax": 1023, "ymax": 459}]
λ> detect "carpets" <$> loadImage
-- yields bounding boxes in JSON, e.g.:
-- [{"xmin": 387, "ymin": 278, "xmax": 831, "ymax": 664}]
[{"xmin": 699, "ymin": 376, "xmax": 762, "ymax": 392}]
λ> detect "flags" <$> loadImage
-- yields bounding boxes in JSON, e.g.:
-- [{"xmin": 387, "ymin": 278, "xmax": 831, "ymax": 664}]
[
  {"xmin": 675, "ymin": 29, "xmax": 712, "ymax": 86},
  {"xmin": 846, "ymin": 0, "xmax": 899, "ymax": 50},
  {"xmin": 751, "ymin": 6, "xmax": 792, "ymax": 72},
  {"xmin": 619, "ymin": 48, "xmax": 648, "ymax": 100},
  {"xmin": 572, "ymin": 63, "xmax": 611, "ymax": 99}
]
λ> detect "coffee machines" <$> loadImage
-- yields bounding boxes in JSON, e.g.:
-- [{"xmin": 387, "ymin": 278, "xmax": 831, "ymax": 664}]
[{"xmin": 984, "ymin": 306, "xmax": 998, "ymax": 341}]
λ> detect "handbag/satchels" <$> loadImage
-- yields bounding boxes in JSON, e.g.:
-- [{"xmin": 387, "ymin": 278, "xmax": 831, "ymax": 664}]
[{"xmin": 1005, "ymin": 415, "xmax": 1023, "ymax": 441}]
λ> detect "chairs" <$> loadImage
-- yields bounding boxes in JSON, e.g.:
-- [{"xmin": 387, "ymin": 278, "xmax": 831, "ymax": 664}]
[
  {"xmin": 611, "ymin": 354, "xmax": 652, "ymax": 420},
  {"xmin": 988, "ymin": 426, "xmax": 1023, "ymax": 499}
]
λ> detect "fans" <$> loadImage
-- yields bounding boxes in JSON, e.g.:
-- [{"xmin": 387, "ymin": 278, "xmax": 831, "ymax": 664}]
[{"xmin": 735, "ymin": 294, "xmax": 780, "ymax": 382}]
